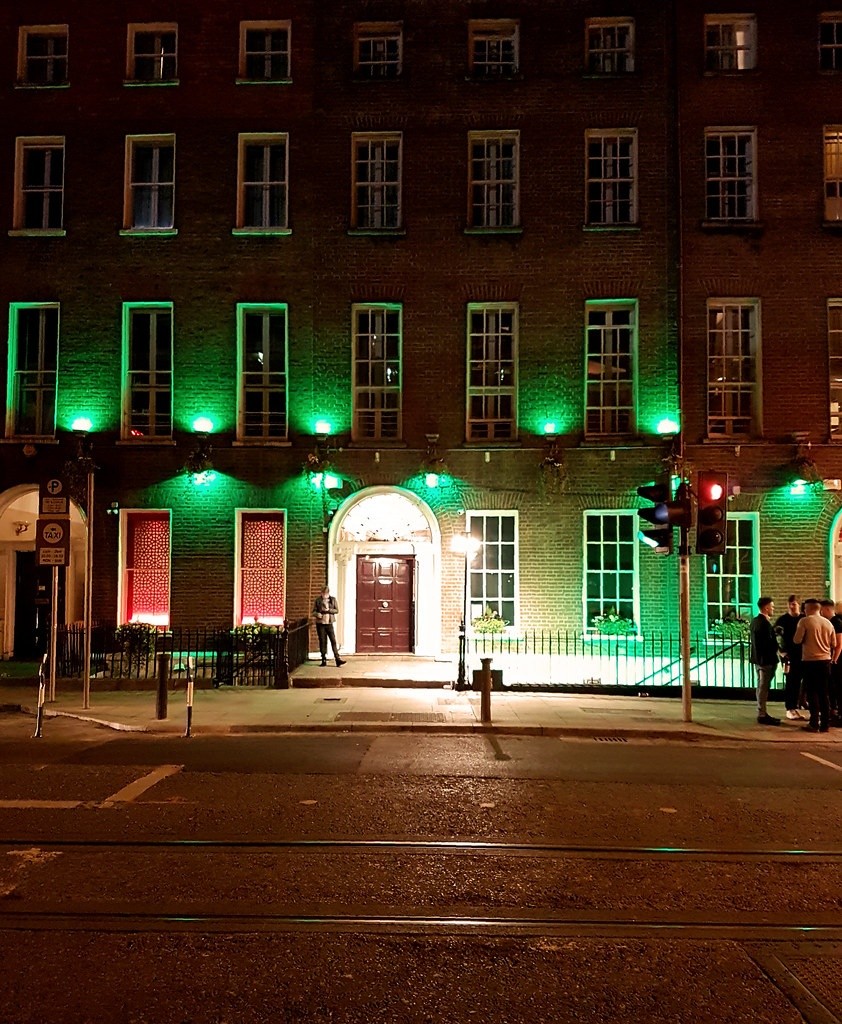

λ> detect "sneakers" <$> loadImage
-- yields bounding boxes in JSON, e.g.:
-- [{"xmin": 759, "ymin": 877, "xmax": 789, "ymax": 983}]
[{"xmin": 786, "ymin": 709, "xmax": 805, "ymax": 720}]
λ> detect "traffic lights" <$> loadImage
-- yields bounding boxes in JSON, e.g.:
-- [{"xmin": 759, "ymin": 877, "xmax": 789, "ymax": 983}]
[
  {"xmin": 693, "ymin": 471, "xmax": 730, "ymax": 555},
  {"xmin": 659, "ymin": 483, "xmax": 692, "ymax": 529},
  {"xmin": 636, "ymin": 472, "xmax": 674, "ymax": 558}
]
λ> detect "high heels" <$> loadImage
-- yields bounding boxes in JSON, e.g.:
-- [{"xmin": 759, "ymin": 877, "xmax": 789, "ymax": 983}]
[{"xmin": 799, "ymin": 698, "xmax": 809, "ymax": 710}]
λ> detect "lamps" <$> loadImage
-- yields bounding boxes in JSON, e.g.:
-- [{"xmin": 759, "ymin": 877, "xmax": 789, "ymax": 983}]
[
  {"xmin": 543, "ymin": 432, "xmax": 559, "ymax": 444},
  {"xmin": 315, "ymin": 432, "xmax": 329, "ymax": 441},
  {"xmin": 788, "ymin": 472, "xmax": 807, "ymax": 487},
  {"xmin": 425, "ymin": 467, "xmax": 439, "ymax": 478},
  {"xmin": 660, "ymin": 431, "xmax": 675, "ymax": 442},
  {"xmin": 72, "ymin": 429, "xmax": 89, "ymax": 441},
  {"xmin": 194, "ymin": 430, "xmax": 209, "ymax": 442}
]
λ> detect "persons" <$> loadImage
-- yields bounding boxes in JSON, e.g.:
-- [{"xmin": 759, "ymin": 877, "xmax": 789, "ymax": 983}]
[
  {"xmin": 312, "ymin": 587, "xmax": 346, "ymax": 667},
  {"xmin": 750, "ymin": 594, "xmax": 842, "ymax": 732}
]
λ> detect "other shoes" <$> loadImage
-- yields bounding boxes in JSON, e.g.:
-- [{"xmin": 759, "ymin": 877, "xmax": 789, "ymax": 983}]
[
  {"xmin": 829, "ymin": 710, "xmax": 841, "ymax": 726},
  {"xmin": 319, "ymin": 659, "xmax": 326, "ymax": 666},
  {"xmin": 336, "ymin": 659, "xmax": 346, "ymax": 667},
  {"xmin": 819, "ymin": 726, "xmax": 829, "ymax": 732},
  {"xmin": 801, "ymin": 724, "xmax": 818, "ymax": 732},
  {"xmin": 758, "ymin": 714, "xmax": 780, "ymax": 725}
]
